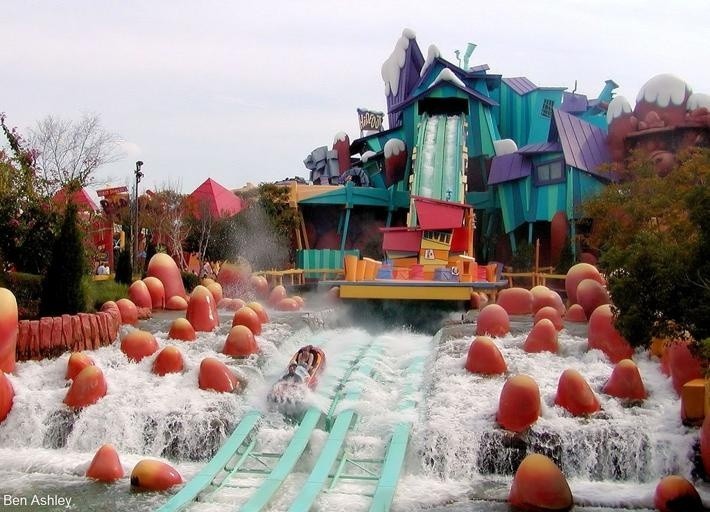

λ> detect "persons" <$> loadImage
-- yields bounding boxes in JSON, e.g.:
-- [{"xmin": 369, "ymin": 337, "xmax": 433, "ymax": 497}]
[
  {"xmin": 95, "ymin": 263, "xmax": 109, "ymax": 274},
  {"xmin": 283, "ymin": 364, "xmax": 303, "ymax": 384},
  {"xmin": 298, "ymin": 345, "xmax": 314, "ymax": 371}
]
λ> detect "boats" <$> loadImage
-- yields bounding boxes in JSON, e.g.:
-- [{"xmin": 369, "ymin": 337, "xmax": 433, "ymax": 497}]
[{"xmin": 267, "ymin": 344, "xmax": 325, "ymax": 402}]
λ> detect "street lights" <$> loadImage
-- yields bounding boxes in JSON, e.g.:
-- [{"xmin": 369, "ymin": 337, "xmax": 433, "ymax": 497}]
[{"xmin": 134, "ymin": 160, "xmax": 144, "ymax": 272}]
